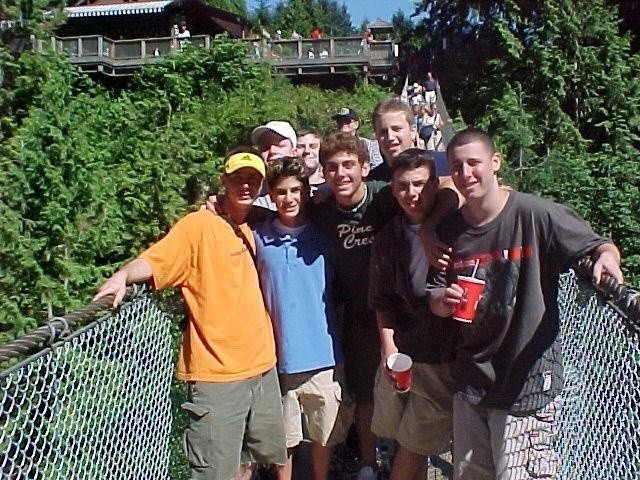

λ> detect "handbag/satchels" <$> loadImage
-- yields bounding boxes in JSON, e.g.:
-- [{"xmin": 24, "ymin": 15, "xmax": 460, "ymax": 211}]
[{"xmin": 420, "ymin": 126, "xmax": 433, "ymax": 139}]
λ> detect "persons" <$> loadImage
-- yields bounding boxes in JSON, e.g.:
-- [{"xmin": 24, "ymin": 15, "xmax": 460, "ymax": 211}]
[
  {"xmin": 203, "ymin": 133, "xmax": 471, "ymax": 478},
  {"xmin": 426, "ymin": 128, "xmax": 626, "ymax": 479},
  {"xmin": 274, "ymin": 28, "xmax": 324, "ymax": 40},
  {"xmin": 407, "ymin": 56, "xmax": 443, "ymax": 147},
  {"xmin": 205, "ymin": 98, "xmax": 449, "ymax": 212},
  {"xmin": 366, "ymin": 148, "xmax": 455, "ymax": 479},
  {"xmin": 174, "ymin": 24, "xmax": 190, "ymax": 38},
  {"xmin": 246, "ymin": 157, "xmax": 359, "ymax": 478},
  {"xmin": 92, "ymin": 146, "xmax": 283, "ymax": 478},
  {"xmin": 360, "ymin": 27, "xmax": 373, "ymax": 49}
]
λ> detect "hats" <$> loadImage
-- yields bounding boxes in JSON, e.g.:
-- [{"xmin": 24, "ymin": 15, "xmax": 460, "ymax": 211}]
[
  {"xmin": 222, "ymin": 153, "xmax": 266, "ymax": 179},
  {"xmin": 251, "ymin": 121, "xmax": 296, "ymax": 149},
  {"xmin": 331, "ymin": 108, "xmax": 358, "ymax": 122}
]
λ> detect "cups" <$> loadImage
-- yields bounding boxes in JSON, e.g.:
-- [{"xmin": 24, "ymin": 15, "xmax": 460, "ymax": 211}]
[
  {"xmin": 453, "ymin": 276, "xmax": 485, "ymax": 322},
  {"xmin": 386, "ymin": 352, "xmax": 415, "ymax": 396}
]
